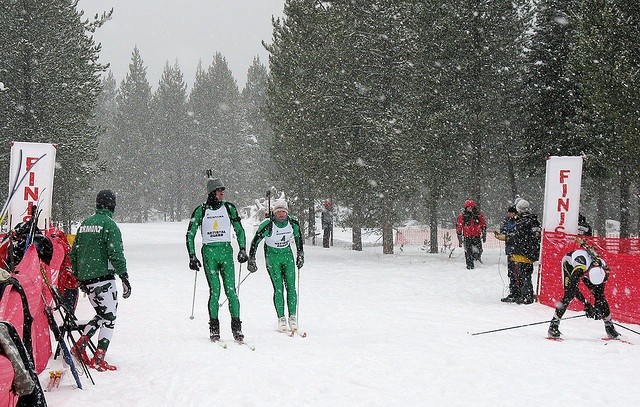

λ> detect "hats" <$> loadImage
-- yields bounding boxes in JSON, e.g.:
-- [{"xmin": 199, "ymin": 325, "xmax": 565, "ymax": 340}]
[
  {"xmin": 273, "ymin": 200, "xmax": 289, "ymax": 214},
  {"xmin": 516, "ymin": 199, "xmax": 530, "ymax": 212},
  {"xmin": 96, "ymin": 189, "xmax": 116, "ymax": 213}
]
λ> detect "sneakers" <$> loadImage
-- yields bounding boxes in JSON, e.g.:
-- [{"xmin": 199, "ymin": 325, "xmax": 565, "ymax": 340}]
[
  {"xmin": 70, "ymin": 335, "xmax": 91, "ymax": 365},
  {"xmin": 501, "ymin": 294, "xmax": 520, "ymax": 302},
  {"xmin": 517, "ymin": 294, "xmax": 534, "ymax": 304},
  {"xmin": 90, "ymin": 349, "xmax": 117, "ymax": 372}
]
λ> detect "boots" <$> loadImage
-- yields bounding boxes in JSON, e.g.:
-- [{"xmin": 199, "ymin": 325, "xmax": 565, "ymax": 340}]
[
  {"xmin": 605, "ymin": 321, "xmax": 621, "ymax": 340},
  {"xmin": 288, "ymin": 315, "xmax": 298, "ymax": 332},
  {"xmin": 278, "ymin": 316, "xmax": 287, "ymax": 332},
  {"xmin": 548, "ymin": 317, "xmax": 563, "ymax": 338},
  {"xmin": 231, "ymin": 319, "xmax": 244, "ymax": 340},
  {"xmin": 209, "ymin": 319, "xmax": 220, "ymax": 340}
]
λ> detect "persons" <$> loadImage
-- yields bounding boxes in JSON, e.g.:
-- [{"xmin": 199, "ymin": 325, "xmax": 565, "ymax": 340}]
[
  {"xmin": 69, "ymin": 189, "xmax": 131, "ymax": 371},
  {"xmin": 578, "ymin": 213, "xmax": 592, "ymax": 236},
  {"xmin": 185, "ymin": 176, "xmax": 248, "ymax": 341},
  {"xmin": 249, "ymin": 199, "xmax": 304, "ymax": 331},
  {"xmin": 547, "ymin": 237, "xmax": 620, "ymax": 339},
  {"xmin": 456, "ymin": 200, "xmax": 487, "ymax": 269},
  {"xmin": 505, "ymin": 199, "xmax": 541, "ymax": 305},
  {"xmin": 321, "ymin": 200, "xmax": 334, "ymax": 248},
  {"xmin": 500, "ymin": 205, "xmax": 518, "ymax": 303}
]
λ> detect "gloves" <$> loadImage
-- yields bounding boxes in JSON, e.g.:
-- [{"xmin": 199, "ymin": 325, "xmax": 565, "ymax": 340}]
[
  {"xmin": 237, "ymin": 248, "xmax": 248, "ymax": 263},
  {"xmin": 119, "ymin": 272, "xmax": 131, "ymax": 299},
  {"xmin": 587, "ymin": 304, "xmax": 595, "ymax": 318},
  {"xmin": 296, "ymin": 251, "xmax": 304, "ymax": 269},
  {"xmin": 189, "ymin": 255, "xmax": 201, "ymax": 271},
  {"xmin": 482, "ymin": 227, "xmax": 486, "ymax": 243},
  {"xmin": 248, "ymin": 259, "xmax": 257, "ymax": 272},
  {"xmin": 595, "ymin": 302, "xmax": 605, "ymax": 319},
  {"xmin": 457, "ymin": 231, "xmax": 462, "ymax": 247},
  {"xmin": 78, "ymin": 281, "xmax": 88, "ymax": 293}
]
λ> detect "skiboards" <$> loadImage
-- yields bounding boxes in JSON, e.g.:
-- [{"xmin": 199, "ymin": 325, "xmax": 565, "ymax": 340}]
[
  {"xmin": 1, "ymin": 149, "xmax": 47, "ymax": 225},
  {"xmin": 213, "ymin": 336, "xmax": 256, "ymax": 351},
  {"xmin": 276, "ymin": 328, "xmax": 308, "ymax": 338},
  {"xmin": 542, "ymin": 334, "xmax": 635, "ymax": 348}
]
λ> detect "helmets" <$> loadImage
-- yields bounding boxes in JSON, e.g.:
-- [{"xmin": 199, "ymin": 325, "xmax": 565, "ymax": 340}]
[
  {"xmin": 464, "ymin": 199, "xmax": 476, "ymax": 209},
  {"xmin": 588, "ymin": 267, "xmax": 606, "ymax": 285},
  {"xmin": 206, "ymin": 177, "xmax": 225, "ymax": 194}
]
